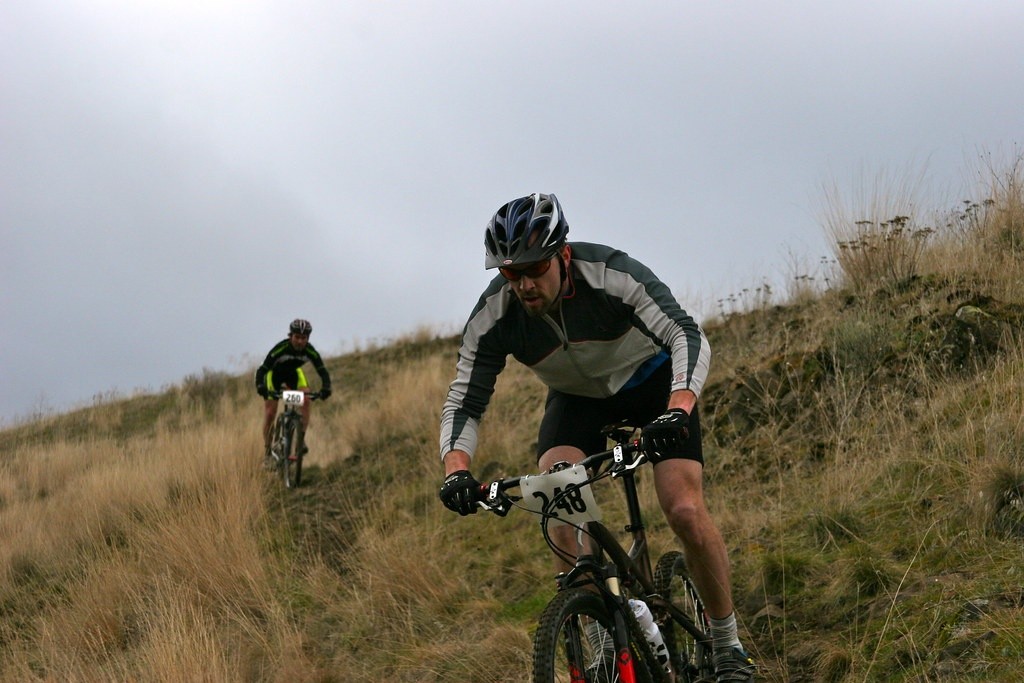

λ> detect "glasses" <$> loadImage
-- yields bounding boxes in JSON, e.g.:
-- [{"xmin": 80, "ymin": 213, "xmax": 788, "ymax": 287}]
[{"xmin": 498, "ymin": 251, "xmax": 558, "ymax": 281}]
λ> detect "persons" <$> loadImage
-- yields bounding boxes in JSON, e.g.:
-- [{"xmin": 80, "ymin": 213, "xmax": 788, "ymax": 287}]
[
  {"xmin": 255, "ymin": 318, "xmax": 331, "ymax": 473},
  {"xmin": 440, "ymin": 194, "xmax": 759, "ymax": 683}
]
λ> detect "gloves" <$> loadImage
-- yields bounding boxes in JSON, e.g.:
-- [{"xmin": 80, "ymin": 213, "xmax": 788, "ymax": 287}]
[
  {"xmin": 440, "ymin": 469, "xmax": 487, "ymax": 517},
  {"xmin": 319, "ymin": 388, "xmax": 333, "ymax": 400},
  {"xmin": 256, "ymin": 385, "xmax": 268, "ymax": 399},
  {"xmin": 638, "ymin": 407, "xmax": 692, "ymax": 452}
]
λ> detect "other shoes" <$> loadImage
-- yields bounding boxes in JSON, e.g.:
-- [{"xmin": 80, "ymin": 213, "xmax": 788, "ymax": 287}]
[
  {"xmin": 261, "ymin": 456, "xmax": 270, "ymax": 471},
  {"xmin": 295, "ymin": 443, "xmax": 308, "ymax": 454}
]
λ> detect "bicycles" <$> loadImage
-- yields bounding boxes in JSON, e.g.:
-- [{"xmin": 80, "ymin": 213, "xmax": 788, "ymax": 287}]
[
  {"xmin": 474, "ymin": 423, "xmax": 716, "ymax": 683},
  {"xmin": 264, "ymin": 388, "xmax": 322, "ymax": 489}
]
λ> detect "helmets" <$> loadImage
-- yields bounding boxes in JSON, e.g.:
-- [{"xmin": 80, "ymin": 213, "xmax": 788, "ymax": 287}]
[
  {"xmin": 482, "ymin": 193, "xmax": 570, "ymax": 270},
  {"xmin": 290, "ymin": 320, "xmax": 312, "ymax": 335}
]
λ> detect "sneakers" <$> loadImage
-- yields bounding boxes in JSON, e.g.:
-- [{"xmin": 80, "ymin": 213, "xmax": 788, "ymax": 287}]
[
  {"xmin": 711, "ymin": 646, "xmax": 755, "ymax": 683},
  {"xmin": 586, "ymin": 646, "xmax": 616, "ymax": 683}
]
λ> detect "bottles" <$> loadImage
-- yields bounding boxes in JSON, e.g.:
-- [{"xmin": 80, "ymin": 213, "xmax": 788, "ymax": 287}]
[{"xmin": 628, "ymin": 598, "xmax": 669, "ymax": 667}]
